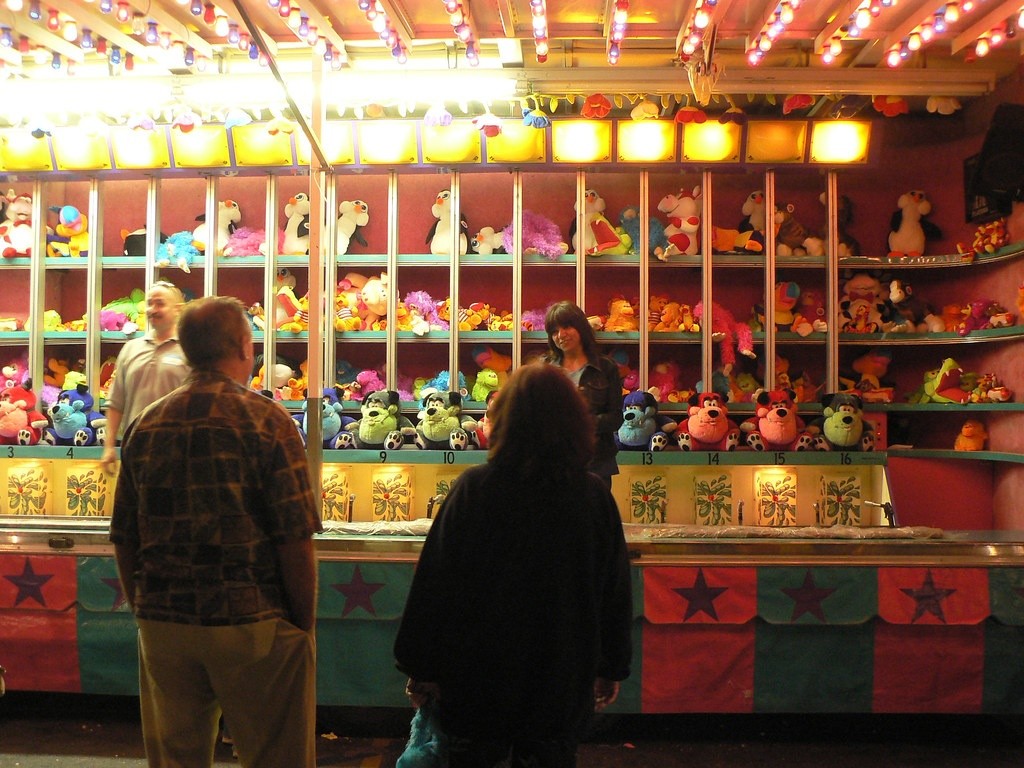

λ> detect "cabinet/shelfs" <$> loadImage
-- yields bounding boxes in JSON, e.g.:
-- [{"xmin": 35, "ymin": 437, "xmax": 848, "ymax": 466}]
[{"xmin": 0, "ymin": 162, "xmax": 1024, "ymax": 469}]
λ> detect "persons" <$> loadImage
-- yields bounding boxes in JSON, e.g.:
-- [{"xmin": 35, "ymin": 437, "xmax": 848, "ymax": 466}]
[
  {"xmin": 394, "ymin": 365, "xmax": 633, "ymax": 768},
  {"xmin": 108, "ymin": 295, "xmax": 325, "ymax": 768},
  {"xmin": 535, "ymin": 300, "xmax": 624, "ymax": 495},
  {"xmin": 103, "ymin": 283, "xmax": 196, "ymax": 474}
]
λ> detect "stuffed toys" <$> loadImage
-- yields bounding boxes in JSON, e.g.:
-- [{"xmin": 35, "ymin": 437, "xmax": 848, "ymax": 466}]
[{"xmin": 0, "ymin": 47, "xmax": 1016, "ymax": 456}]
